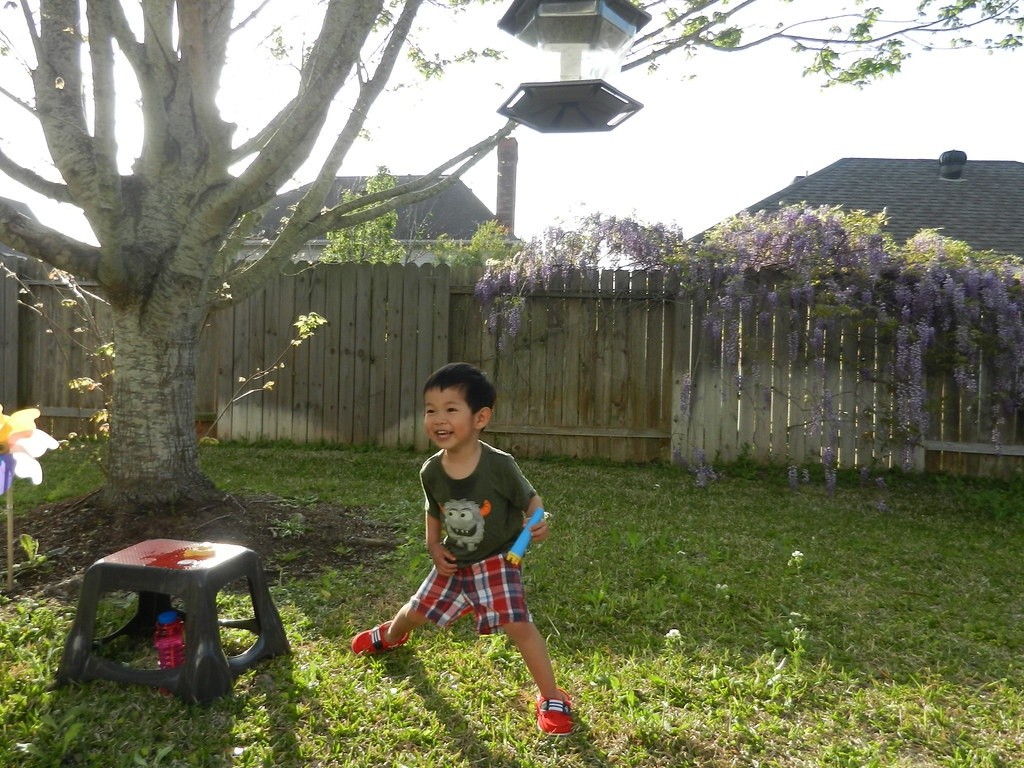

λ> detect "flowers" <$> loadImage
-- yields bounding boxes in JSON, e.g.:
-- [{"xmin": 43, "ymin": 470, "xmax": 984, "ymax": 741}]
[{"xmin": 0, "ymin": 404, "xmax": 59, "ymax": 496}]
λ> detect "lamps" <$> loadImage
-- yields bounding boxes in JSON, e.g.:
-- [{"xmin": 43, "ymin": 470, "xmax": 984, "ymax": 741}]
[
  {"xmin": 496, "ymin": 77, "xmax": 646, "ymax": 135},
  {"xmin": 496, "ymin": 0, "xmax": 652, "ymax": 52}
]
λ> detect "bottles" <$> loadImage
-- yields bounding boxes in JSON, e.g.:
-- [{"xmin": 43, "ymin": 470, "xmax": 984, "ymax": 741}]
[{"xmin": 154, "ymin": 612, "xmax": 189, "ymax": 696}]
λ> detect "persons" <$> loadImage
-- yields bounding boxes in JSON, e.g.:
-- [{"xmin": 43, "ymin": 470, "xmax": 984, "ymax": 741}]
[{"xmin": 352, "ymin": 362, "xmax": 577, "ymax": 740}]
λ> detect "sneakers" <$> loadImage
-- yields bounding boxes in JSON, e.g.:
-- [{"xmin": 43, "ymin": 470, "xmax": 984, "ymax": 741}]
[
  {"xmin": 352, "ymin": 620, "xmax": 410, "ymax": 655},
  {"xmin": 536, "ymin": 688, "xmax": 573, "ymax": 736}
]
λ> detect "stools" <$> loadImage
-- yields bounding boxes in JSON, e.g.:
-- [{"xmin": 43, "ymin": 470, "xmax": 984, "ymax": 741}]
[{"xmin": 47, "ymin": 537, "xmax": 292, "ymax": 711}]
